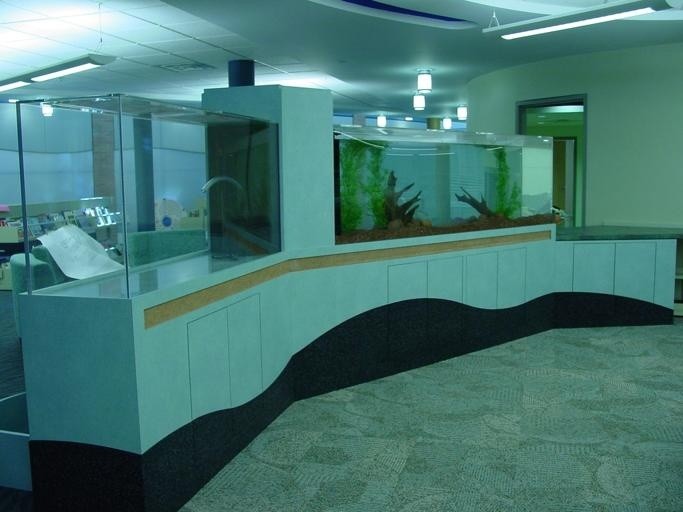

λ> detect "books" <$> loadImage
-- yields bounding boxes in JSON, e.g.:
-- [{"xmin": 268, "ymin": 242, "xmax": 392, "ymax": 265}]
[{"xmin": 0, "ymin": 204, "xmax": 117, "ymax": 244}]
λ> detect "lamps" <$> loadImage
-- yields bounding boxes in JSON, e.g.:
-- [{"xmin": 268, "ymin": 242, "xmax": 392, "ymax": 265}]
[
  {"xmin": 376, "ymin": 68, "xmax": 468, "ymax": 129},
  {"xmin": 41, "ymin": 105, "xmax": 53, "ymax": 116},
  {"xmin": 482, "ymin": 0, "xmax": 674, "ymax": 41},
  {"xmin": 0, "ymin": 0, "xmax": 116, "ymax": 93}
]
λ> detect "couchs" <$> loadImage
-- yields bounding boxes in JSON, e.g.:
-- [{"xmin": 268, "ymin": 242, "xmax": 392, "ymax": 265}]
[{"xmin": 10, "ymin": 228, "xmax": 207, "ymax": 348}]
